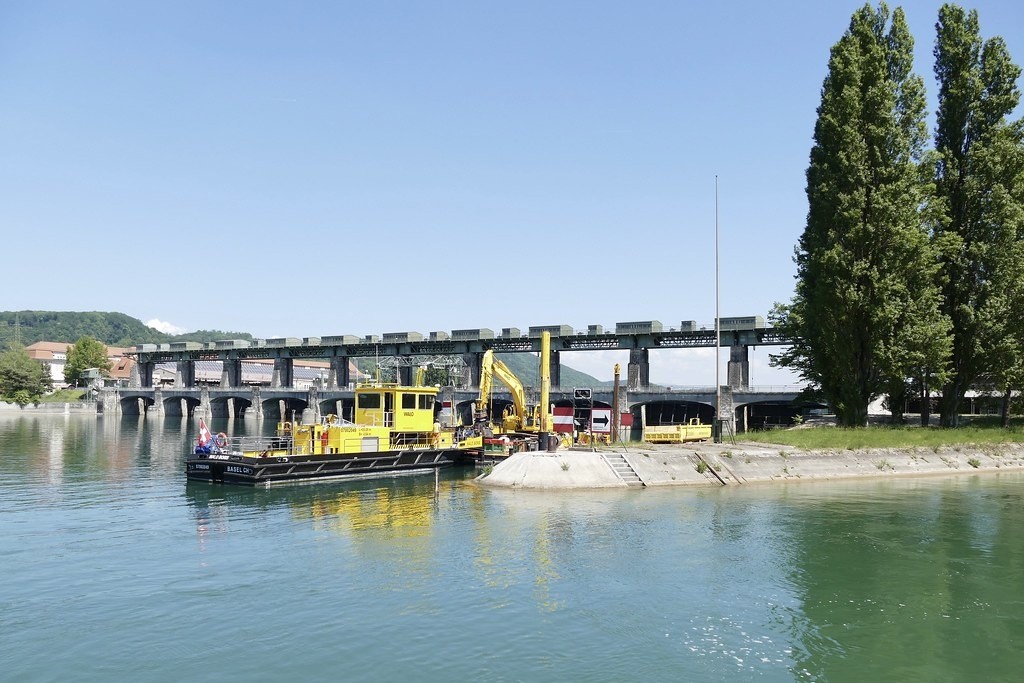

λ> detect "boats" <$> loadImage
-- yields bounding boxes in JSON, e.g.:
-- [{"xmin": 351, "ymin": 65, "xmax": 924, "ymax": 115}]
[{"xmin": 184, "ymin": 362, "xmax": 485, "ymax": 487}]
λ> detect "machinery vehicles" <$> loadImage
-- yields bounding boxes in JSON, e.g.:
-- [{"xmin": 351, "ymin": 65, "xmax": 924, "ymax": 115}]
[{"xmin": 479, "ymin": 349, "xmax": 559, "ymax": 460}]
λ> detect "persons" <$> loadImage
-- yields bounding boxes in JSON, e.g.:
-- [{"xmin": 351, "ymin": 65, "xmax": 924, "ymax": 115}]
[
  {"xmin": 199, "ymin": 421, "xmax": 212, "ymax": 448},
  {"xmin": 431, "ymin": 419, "xmax": 440, "ymax": 448}
]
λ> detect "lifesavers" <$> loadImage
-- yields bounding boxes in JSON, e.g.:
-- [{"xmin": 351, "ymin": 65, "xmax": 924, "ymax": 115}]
[{"xmin": 213, "ymin": 432, "xmax": 228, "ymax": 449}]
